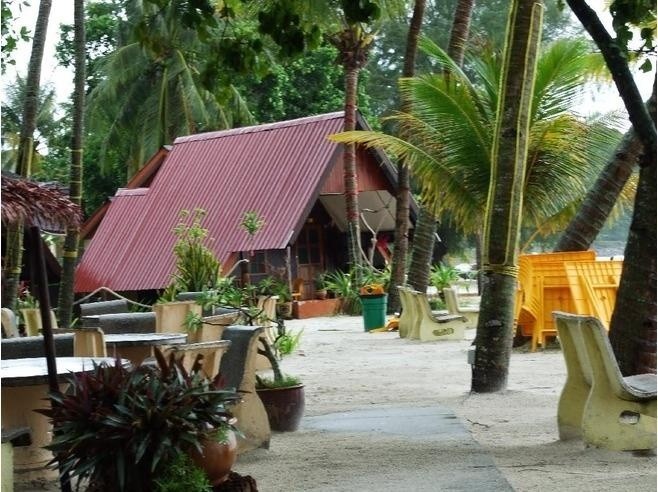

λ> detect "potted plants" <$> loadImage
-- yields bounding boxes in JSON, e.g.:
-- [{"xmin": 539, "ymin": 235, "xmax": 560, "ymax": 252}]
[
  {"xmin": 188, "ymin": 279, "xmax": 306, "ymax": 430},
  {"xmin": 313, "ymin": 270, "xmax": 328, "ymax": 300}
]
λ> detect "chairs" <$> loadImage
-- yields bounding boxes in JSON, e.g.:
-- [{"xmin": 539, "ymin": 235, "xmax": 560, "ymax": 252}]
[
  {"xmin": 552, "ymin": 308, "xmax": 657, "ymax": 451},
  {"xmin": 0, "ymin": 294, "xmax": 281, "ymax": 492},
  {"xmin": 397, "ymin": 286, "xmax": 482, "ymax": 342}
]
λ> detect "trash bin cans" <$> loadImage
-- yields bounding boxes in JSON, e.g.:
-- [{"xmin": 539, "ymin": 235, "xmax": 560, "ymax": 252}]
[{"xmin": 359, "ymin": 293, "xmax": 388, "ymax": 332}]
